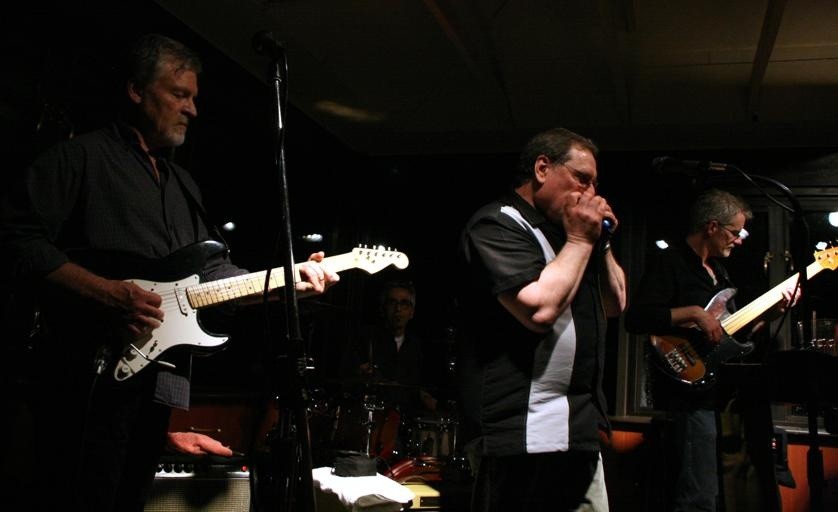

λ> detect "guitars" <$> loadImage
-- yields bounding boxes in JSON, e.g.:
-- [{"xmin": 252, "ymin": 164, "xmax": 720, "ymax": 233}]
[
  {"xmin": 649, "ymin": 239, "xmax": 838, "ymax": 381},
  {"xmin": 64, "ymin": 238, "xmax": 410, "ymax": 413}
]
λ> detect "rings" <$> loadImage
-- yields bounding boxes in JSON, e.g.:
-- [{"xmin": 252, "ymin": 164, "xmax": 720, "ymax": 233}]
[{"xmin": 319, "ymin": 277, "xmax": 326, "ymax": 281}]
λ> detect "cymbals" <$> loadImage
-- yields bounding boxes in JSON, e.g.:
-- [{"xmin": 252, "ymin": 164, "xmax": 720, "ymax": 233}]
[{"xmin": 356, "ymin": 377, "xmax": 419, "ymax": 390}]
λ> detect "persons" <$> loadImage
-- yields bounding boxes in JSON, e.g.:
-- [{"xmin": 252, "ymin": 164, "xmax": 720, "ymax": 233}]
[
  {"xmin": 1, "ymin": 27, "xmax": 343, "ymax": 511},
  {"xmin": 442, "ymin": 125, "xmax": 627, "ymax": 511},
  {"xmin": 344, "ymin": 275, "xmax": 445, "ymax": 414},
  {"xmin": 627, "ymin": 188, "xmax": 805, "ymax": 511}
]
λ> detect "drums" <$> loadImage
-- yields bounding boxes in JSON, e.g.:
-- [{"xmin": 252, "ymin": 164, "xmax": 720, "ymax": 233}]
[
  {"xmin": 323, "ymin": 388, "xmax": 406, "ymax": 466},
  {"xmin": 405, "ymin": 417, "xmax": 459, "ymax": 461}
]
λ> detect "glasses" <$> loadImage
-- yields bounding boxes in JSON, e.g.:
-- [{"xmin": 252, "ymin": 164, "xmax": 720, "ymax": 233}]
[
  {"xmin": 719, "ymin": 224, "xmax": 740, "ymax": 236},
  {"xmin": 557, "ymin": 158, "xmax": 599, "ymax": 189}
]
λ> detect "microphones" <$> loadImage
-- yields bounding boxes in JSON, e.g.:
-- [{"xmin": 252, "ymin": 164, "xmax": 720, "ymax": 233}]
[
  {"xmin": 243, "ymin": 30, "xmax": 293, "ymax": 79},
  {"xmin": 650, "ymin": 154, "xmax": 738, "ymax": 175}
]
